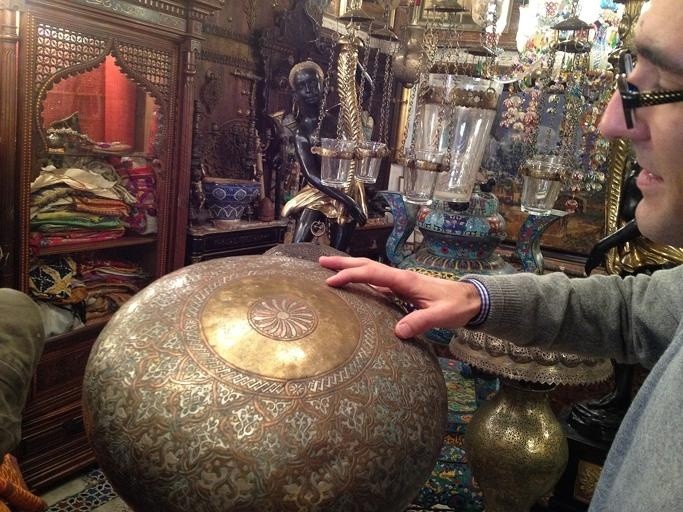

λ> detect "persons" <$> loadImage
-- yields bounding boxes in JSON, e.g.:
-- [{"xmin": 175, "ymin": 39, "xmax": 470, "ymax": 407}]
[
  {"xmin": 286, "ymin": 62, "xmax": 369, "ymax": 249},
  {"xmin": 315, "ymin": 1, "xmax": 682, "ymax": 510}
]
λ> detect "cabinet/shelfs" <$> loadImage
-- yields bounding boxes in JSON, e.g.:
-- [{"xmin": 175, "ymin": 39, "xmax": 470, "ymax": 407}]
[{"xmin": 0, "ymin": 0, "xmax": 225, "ymax": 497}]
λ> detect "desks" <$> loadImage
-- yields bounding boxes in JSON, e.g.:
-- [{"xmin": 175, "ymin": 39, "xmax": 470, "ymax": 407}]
[{"xmin": 187, "ymin": 219, "xmax": 396, "ymax": 267}]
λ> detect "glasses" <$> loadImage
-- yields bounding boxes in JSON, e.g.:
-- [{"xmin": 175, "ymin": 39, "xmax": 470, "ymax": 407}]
[{"xmin": 612, "ymin": 49, "xmax": 679, "ymax": 131}]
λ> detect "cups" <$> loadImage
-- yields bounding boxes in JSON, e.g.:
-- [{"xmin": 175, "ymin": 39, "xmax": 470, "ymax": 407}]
[
  {"xmin": 321, "ymin": 138, "xmax": 443, "ymax": 201},
  {"xmin": 519, "ymin": 153, "xmax": 565, "ymax": 216},
  {"xmin": 423, "ymin": 73, "xmax": 505, "ymax": 204}
]
